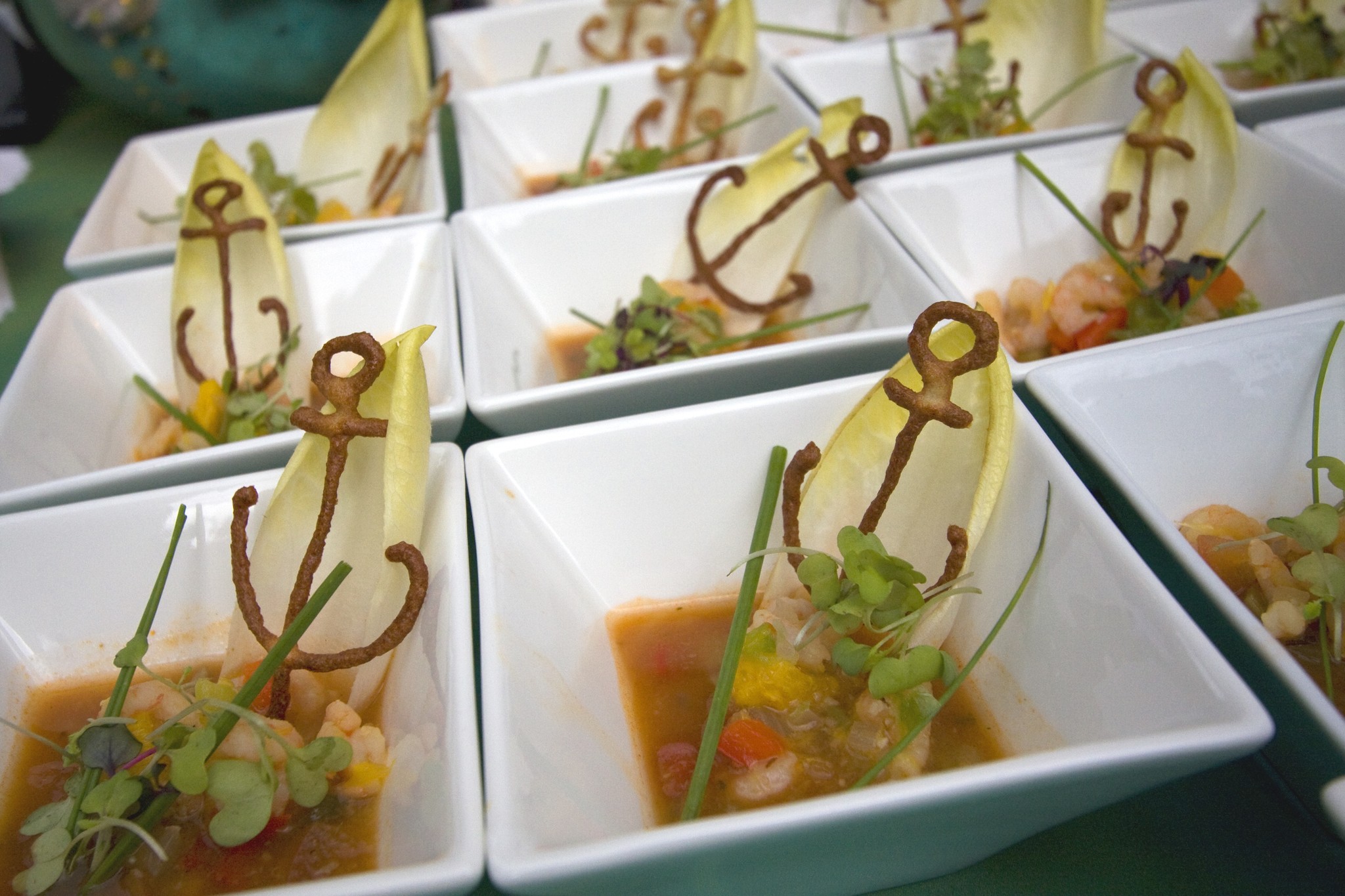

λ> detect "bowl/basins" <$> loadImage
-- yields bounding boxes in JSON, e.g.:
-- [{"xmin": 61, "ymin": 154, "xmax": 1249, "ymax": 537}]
[
  {"xmin": 778, "ymin": 21, "xmax": 1167, "ymax": 172},
  {"xmin": 427, "ymin": 0, "xmax": 712, "ymax": 101},
  {"xmin": 452, "ymin": 55, "xmax": 821, "ymax": 212},
  {"xmin": 63, "ymin": 103, "xmax": 448, "ymax": 279},
  {"xmin": 447, "ymin": 174, "xmax": 954, "ymax": 437},
  {"xmin": 716, "ymin": 0, "xmax": 989, "ymax": 63},
  {"xmin": 0, "ymin": 441, "xmax": 485, "ymax": 896},
  {"xmin": 0, "ymin": 220, "xmax": 467, "ymax": 515},
  {"xmin": 465, "ymin": 370, "xmax": 1274, "ymax": 896},
  {"xmin": 1025, "ymin": 304, "xmax": 1345, "ymax": 755},
  {"xmin": 853, "ymin": 120, "xmax": 1345, "ymax": 384},
  {"xmin": 1256, "ymin": 107, "xmax": 1345, "ymax": 178},
  {"xmin": 1103, "ymin": 1, "xmax": 1344, "ymax": 129}
]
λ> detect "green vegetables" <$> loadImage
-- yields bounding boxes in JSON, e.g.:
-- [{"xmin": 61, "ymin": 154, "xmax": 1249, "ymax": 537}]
[
  {"xmin": 678, "ymin": 444, "xmax": 1051, "ymax": 819},
  {"xmin": 135, "ymin": 325, "xmax": 303, "ymax": 446},
  {"xmin": 531, "ymin": 24, "xmax": 852, "ymax": 185},
  {"xmin": 1266, "ymin": 321, "xmax": 1345, "ymax": 708},
  {"xmin": 888, "ymin": 34, "xmax": 1137, "ymax": 146},
  {"xmin": 135, "ymin": 141, "xmax": 362, "ymax": 227},
  {"xmin": 1012, "ymin": 151, "xmax": 1267, "ymax": 342},
  {"xmin": 1213, "ymin": 14, "xmax": 1330, "ymax": 83},
  {"xmin": 570, "ymin": 275, "xmax": 871, "ymax": 378},
  {"xmin": 0, "ymin": 504, "xmax": 353, "ymax": 896}
]
